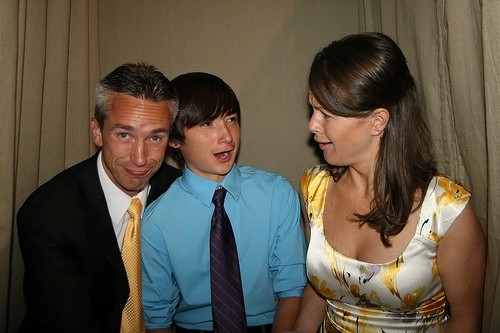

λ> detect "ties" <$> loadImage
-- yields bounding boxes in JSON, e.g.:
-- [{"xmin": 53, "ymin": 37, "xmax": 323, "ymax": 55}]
[
  {"xmin": 209, "ymin": 186, "xmax": 248, "ymax": 333},
  {"xmin": 120, "ymin": 197, "xmax": 147, "ymax": 333}
]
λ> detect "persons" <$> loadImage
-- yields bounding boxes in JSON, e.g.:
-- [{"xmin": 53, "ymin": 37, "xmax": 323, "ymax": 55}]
[
  {"xmin": 139, "ymin": 70, "xmax": 308, "ymax": 333},
  {"xmin": 289, "ymin": 31, "xmax": 488, "ymax": 333},
  {"xmin": 15, "ymin": 62, "xmax": 184, "ymax": 332}
]
